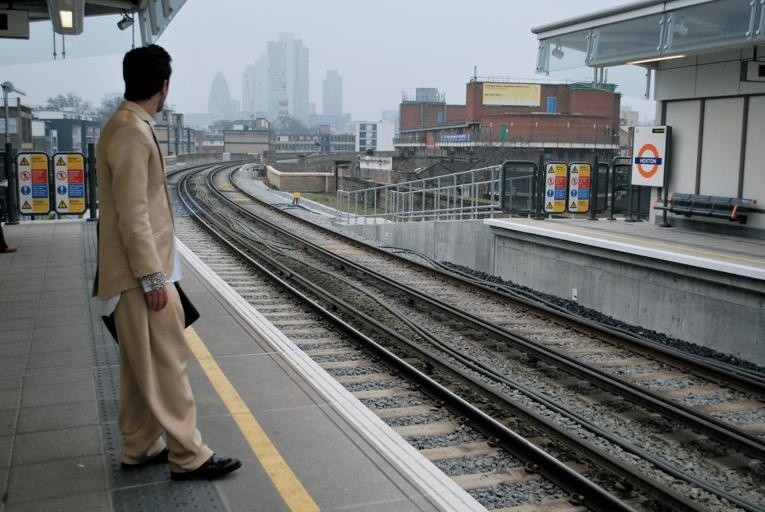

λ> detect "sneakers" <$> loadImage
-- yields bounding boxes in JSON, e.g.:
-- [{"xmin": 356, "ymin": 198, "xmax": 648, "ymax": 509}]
[{"xmin": 0, "ymin": 246, "xmax": 17, "ymax": 253}]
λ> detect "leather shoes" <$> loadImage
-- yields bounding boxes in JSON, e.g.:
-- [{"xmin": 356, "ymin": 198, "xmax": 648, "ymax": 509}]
[{"xmin": 120, "ymin": 446, "xmax": 241, "ymax": 481}]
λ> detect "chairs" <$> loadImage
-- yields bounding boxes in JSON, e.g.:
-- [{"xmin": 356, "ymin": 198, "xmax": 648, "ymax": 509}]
[{"xmin": 654, "ymin": 192, "xmax": 756, "ymax": 224}]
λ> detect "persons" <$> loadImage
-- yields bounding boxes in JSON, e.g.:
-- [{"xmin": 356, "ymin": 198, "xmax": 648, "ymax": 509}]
[
  {"xmin": 0, "ymin": 218, "xmax": 17, "ymax": 254},
  {"xmin": 92, "ymin": 45, "xmax": 243, "ymax": 482}
]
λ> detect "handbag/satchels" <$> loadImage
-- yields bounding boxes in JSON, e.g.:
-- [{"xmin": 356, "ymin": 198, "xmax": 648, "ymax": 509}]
[{"xmin": 102, "ymin": 281, "xmax": 201, "ymax": 346}]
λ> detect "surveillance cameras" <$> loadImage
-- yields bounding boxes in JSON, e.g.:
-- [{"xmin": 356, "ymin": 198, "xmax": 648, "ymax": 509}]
[
  {"xmin": 117, "ymin": 17, "xmax": 134, "ymax": 30},
  {"xmin": 675, "ymin": 24, "xmax": 688, "ymax": 37},
  {"xmin": 552, "ymin": 49, "xmax": 564, "ymax": 59}
]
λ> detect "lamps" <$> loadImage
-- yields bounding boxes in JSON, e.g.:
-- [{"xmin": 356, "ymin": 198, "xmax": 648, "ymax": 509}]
[
  {"xmin": 552, "ymin": 40, "xmax": 564, "ymax": 59},
  {"xmin": 117, "ymin": 8, "xmax": 134, "ymax": 30},
  {"xmin": 673, "ymin": 18, "xmax": 689, "ymax": 36}
]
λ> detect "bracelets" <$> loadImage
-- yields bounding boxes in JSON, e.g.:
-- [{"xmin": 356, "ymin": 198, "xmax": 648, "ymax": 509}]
[{"xmin": 142, "ymin": 273, "xmax": 169, "ymax": 289}]
